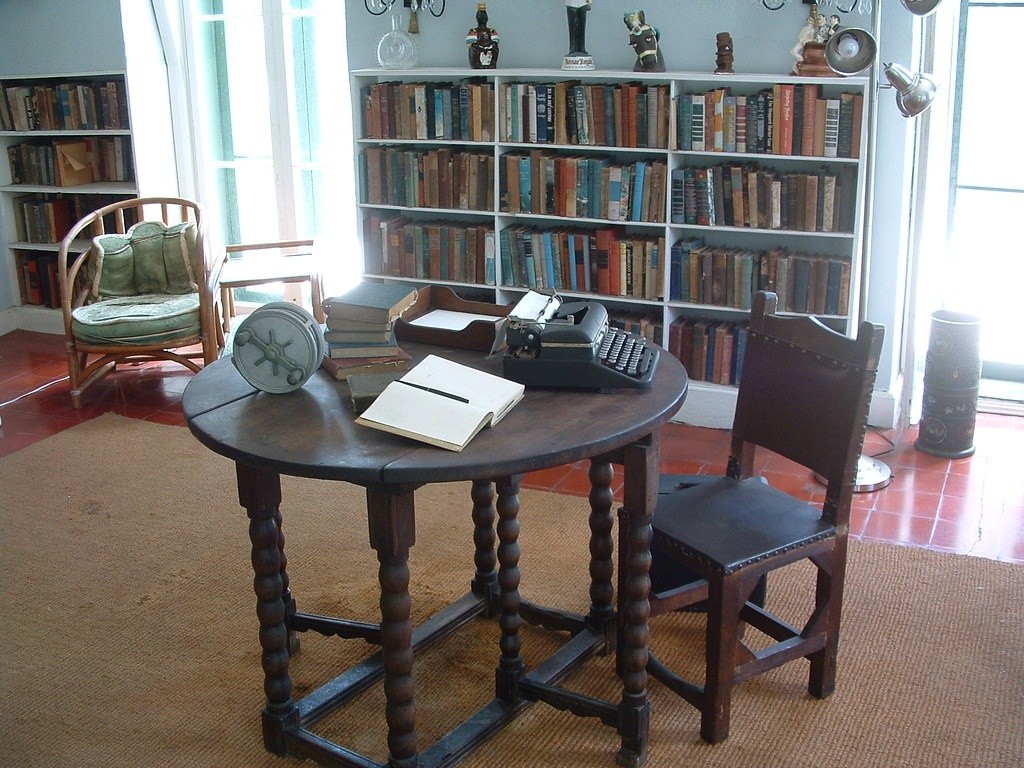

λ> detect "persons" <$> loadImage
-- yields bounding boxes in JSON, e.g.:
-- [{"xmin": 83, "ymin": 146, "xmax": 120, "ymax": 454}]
[{"xmin": 789, "ymin": 15, "xmax": 840, "ymax": 77}]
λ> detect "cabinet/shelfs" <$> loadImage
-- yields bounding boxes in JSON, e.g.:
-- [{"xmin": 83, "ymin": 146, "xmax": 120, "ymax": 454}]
[
  {"xmin": 1, "ymin": 70, "xmax": 146, "ymax": 337},
  {"xmin": 350, "ymin": 69, "xmax": 870, "ymax": 428}
]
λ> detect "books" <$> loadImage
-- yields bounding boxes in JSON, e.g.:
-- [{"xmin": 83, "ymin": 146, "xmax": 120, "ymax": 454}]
[
  {"xmin": 317, "ymin": 278, "xmax": 527, "ymax": 452},
  {"xmin": 359, "ymin": 79, "xmax": 863, "ymax": 387},
  {"xmin": 1, "ymin": 78, "xmax": 137, "ymax": 311}
]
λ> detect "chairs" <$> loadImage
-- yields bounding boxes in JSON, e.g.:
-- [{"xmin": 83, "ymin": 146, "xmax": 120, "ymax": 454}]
[
  {"xmin": 618, "ymin": 289, "xmax": 885, "ymax": 742},
  {"xmin": 57, "ymin": 196, "xmax": 228, "ymax": 411}
]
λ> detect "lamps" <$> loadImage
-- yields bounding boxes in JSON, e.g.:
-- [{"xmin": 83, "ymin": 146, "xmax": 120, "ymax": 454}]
[{"xmin": 814, "ymin": 0, "xmax": 944, "ymax": 492}]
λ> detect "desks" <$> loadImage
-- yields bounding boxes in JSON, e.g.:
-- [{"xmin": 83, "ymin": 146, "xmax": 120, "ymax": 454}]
[
  {"xmin": 208, "ymin": 239, "xmax": 326, "ymax": 362},
  {"xmin": 182, "ymin": 322, "xmax": 688, "ymax": 768}
]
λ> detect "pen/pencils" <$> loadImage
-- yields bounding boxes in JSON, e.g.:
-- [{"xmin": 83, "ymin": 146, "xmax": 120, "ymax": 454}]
[{"xmin": 393, "ymin": 379, "xmax": 470, "ymax": 404}]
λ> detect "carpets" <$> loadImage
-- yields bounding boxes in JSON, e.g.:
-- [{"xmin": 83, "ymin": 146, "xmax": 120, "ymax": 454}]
[{"xmin": 0, "ymin": 411, "xmax": 1023, "ymax": 768}]
